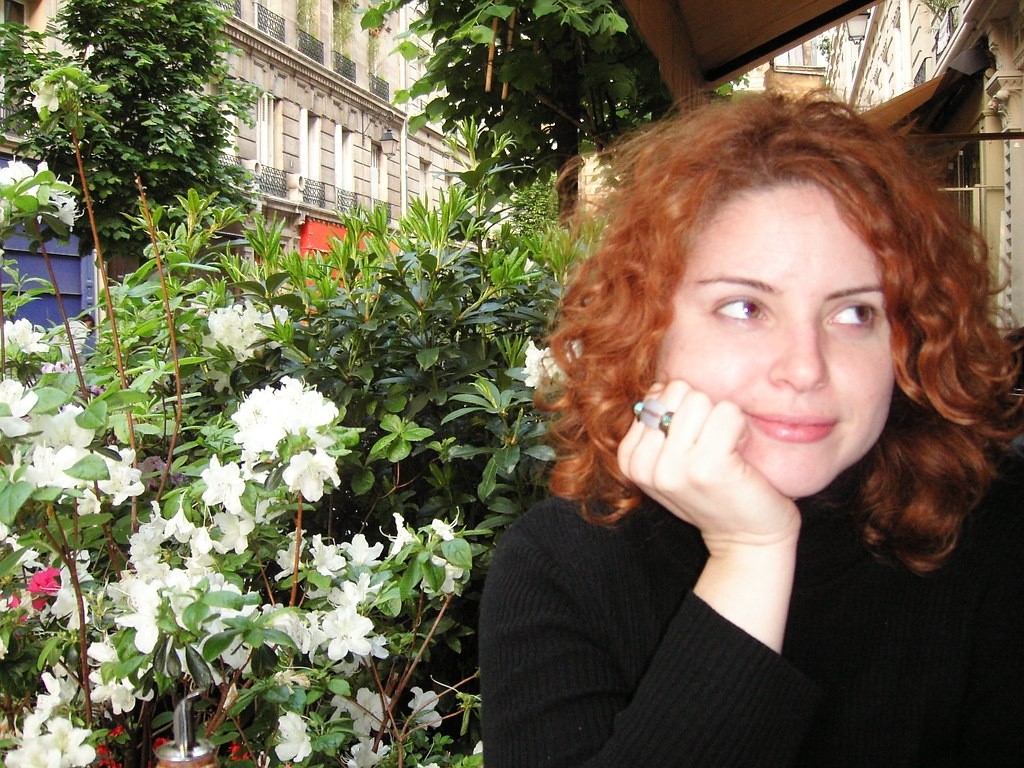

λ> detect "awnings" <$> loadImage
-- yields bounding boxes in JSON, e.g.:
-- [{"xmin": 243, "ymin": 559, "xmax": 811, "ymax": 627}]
[{"xmin": 620, "ymin": 0, "xmax": 884, "ymax": 112}]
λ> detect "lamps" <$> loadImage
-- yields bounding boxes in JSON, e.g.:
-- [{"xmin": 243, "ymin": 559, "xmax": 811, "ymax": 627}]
[
  {"xmin": 845, "ymin": 10, "xmax": 872, "ymax": 44},
  {"xmin": 361, "ymin": 107, "xmax": 401, "ymax": 159}
]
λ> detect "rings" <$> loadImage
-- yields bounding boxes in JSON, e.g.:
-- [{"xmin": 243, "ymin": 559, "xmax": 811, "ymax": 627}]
[{"xmin": 634, "ymin": 400, "xmax": 673, "ymax": 434}]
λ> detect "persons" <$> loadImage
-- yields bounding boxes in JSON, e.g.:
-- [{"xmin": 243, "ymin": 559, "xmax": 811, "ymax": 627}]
[
  {"xmin": 481, "ymin": 92, "xmax": 1024, "ymax": 768},
  {"xmin": 52, "ymin": 310, "xmax": 95, "ymax": 362}
]
